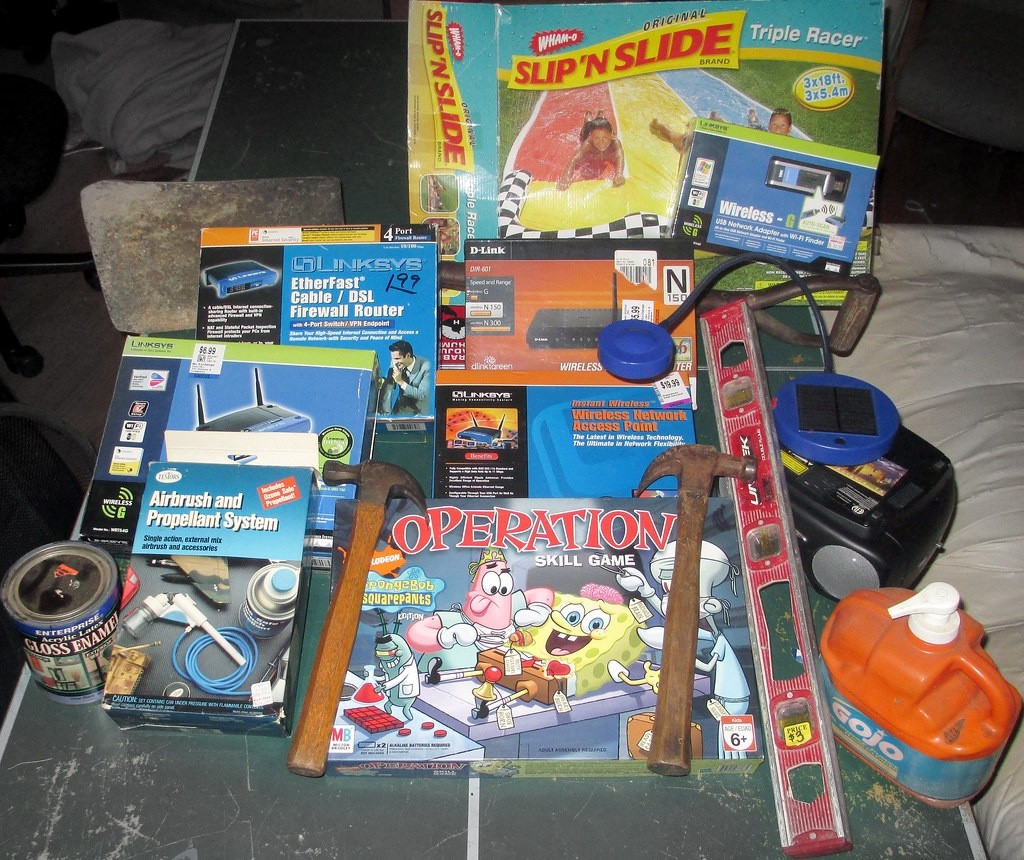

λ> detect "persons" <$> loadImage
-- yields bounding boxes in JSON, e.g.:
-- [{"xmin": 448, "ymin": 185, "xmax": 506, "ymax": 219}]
[
  {"xmin": 711, "ymin": 108, "xmax": 793, "ymax": 138},
  {"xmin": 388, "ymin": 340, "xmax": 431, "ymax": 417},
  {"xmin": 650, "ymin": 118, "xmax": 690, "ymax": 151},
  {"xmin": 558, "ymin": 109, "xmax": 626, "ymax": 191}
]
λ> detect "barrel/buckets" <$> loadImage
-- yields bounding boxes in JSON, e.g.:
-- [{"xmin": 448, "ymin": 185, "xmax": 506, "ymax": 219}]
[{"xmin": 1, "ymin": 539, "xmax": 123, "ymax": 705}]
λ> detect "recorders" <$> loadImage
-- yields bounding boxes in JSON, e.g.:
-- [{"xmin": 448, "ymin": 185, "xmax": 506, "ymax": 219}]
[{"xmin": 776, "ymin": 419, "xmax": 959, "ymax": 604}]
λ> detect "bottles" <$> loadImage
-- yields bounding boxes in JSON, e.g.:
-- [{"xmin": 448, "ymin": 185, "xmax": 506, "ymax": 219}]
[
  {"xmin": 240, "ymin": 564, "xmax": 301, "ymax": 637},
  {"xmin": 817, "ymin": 580, "xmax": 1024, "ymax": 810}
]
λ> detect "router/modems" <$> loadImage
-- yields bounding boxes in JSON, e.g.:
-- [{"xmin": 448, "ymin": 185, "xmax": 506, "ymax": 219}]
[{"xmin": 196, "ymin": 367, "xmax": 311, "ymax": 432}]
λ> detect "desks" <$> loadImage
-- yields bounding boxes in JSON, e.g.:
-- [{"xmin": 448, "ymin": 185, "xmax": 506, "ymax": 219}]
[{"xmin": 0, "ymin": 16, "xmax": 991, "ymax": 860}]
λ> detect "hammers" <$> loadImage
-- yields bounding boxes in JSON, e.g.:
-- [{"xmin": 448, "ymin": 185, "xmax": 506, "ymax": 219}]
[
  {"xmin": 285, "ymin": 455, "xmax": 426, "ymax": 779},
  {"xmin": 636, "ymin": 445, "xmax": 761, "ymax": 779}
]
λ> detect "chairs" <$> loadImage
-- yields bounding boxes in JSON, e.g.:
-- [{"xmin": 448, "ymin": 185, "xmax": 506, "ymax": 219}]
[{"xmin": 0, "ymin": 73, "xmax": 107, "ymax": 378}]
